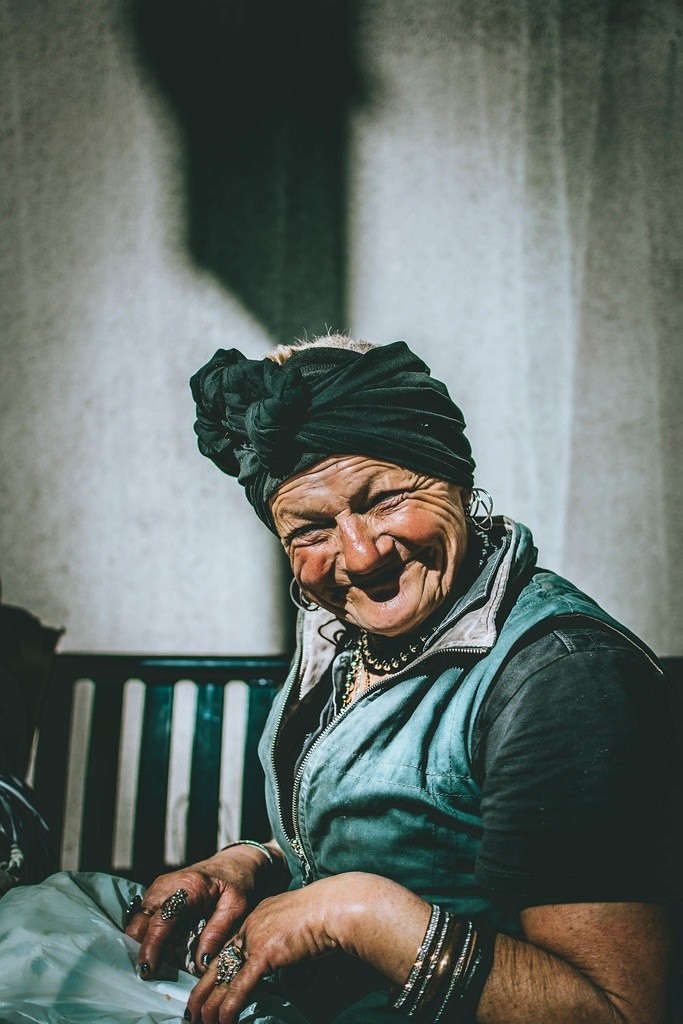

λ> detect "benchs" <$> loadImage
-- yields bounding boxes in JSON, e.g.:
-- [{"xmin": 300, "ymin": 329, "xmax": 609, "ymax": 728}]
[{"xmin": 0, "ymin": 654, "xmax": 683, "ymax": 891}]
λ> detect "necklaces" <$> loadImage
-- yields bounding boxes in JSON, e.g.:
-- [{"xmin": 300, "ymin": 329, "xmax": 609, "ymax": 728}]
[{"xmin": 340, "ymin": 532, "xmax": 498, "ymax": 713}]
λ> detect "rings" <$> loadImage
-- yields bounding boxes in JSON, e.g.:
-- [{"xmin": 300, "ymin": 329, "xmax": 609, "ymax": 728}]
[
  {"xmin": 126, "ymin": 895, "xmax": 154, "ymax": 925},
  {"xmin": 160, "ymin": 889, "xmax": 189, "ymax": 923},
  {"xmin": 214, "ymin": 944, "xmax": 245, "ymax": 984}
]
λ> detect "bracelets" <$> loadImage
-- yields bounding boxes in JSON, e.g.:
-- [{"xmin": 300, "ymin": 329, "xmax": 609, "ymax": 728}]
[
  {"xmin": 388, "ymin": 903, "xmax": 496, "ymax": 1024},
  {"xmin": 220, "ymin": 841, "xmax": 289, "ymax": 892}
]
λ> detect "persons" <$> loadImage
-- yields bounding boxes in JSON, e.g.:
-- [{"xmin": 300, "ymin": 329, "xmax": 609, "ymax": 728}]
[{"xmin": 126, "ymin": 335, "xmax": 683, "ymax": 1024}]
